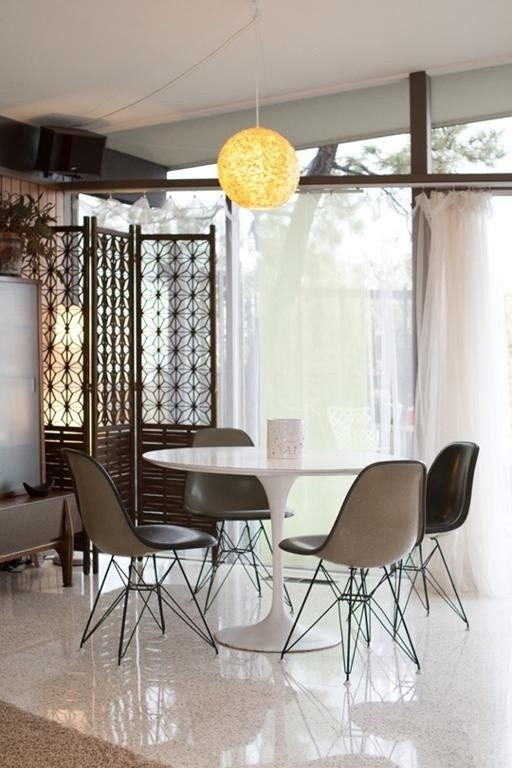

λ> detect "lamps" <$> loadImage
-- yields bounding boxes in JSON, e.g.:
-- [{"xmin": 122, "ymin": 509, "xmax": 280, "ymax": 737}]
[{"xmin": 218, "ymin": 0, "xmax": 301, "ymax": 210}]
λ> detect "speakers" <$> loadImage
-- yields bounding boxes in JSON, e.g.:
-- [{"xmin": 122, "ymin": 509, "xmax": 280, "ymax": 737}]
[{"xmin": 35, "ymin": 124, "xmax": 107, "ymax": 175}]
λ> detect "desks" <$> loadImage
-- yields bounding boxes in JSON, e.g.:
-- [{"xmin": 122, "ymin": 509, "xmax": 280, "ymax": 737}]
[
  {"xmin": 0, "ymin": 491, "xmax": 85, "ymax": 588},
  {"xmin": 140, "ymin": 446, "xmax": 409, "ymax": 652}
]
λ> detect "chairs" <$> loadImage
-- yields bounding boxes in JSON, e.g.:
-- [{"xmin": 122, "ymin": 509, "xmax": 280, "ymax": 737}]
[
  {"xmin": 60, "ymin": 447, "xmax": 219, "ymax": 666},
  {"xmin": 349, "ymin": 440, "xmax": 480, "ymax": 639},
  {"xmin": 278, "ymin": 459, "xmax": 427, "ymax": 682},
  {"xmin": 184, "ymin": 427, "xmax": 294, "ymax": 619}
]
